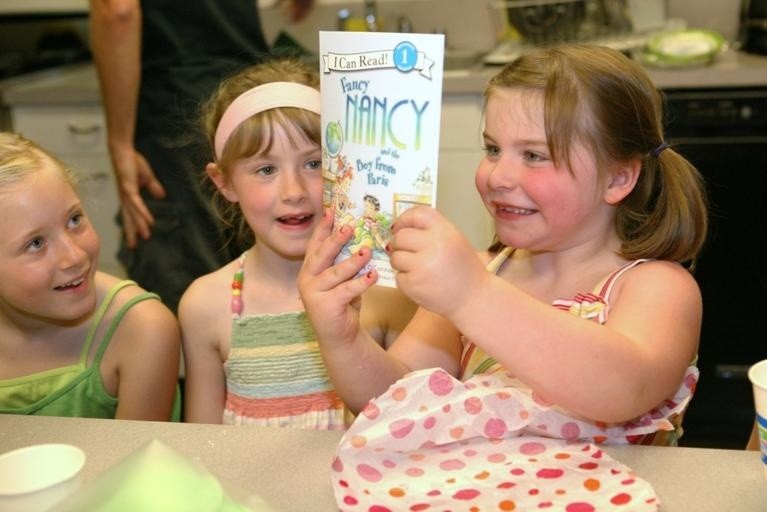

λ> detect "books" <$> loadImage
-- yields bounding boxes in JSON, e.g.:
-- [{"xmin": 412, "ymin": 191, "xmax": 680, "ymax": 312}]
[{"xmin": 319, "ymin": 30, "xmax": 444, "ymax": 288}]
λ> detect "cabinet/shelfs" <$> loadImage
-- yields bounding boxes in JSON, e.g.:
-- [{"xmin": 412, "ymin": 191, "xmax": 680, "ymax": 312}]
[
  {"xmin": 9, "ymin": 93, "xmax": 499, "ymax": 280},
  {"xmin": 654, "ymin": 89, "xmax": 766, "ymax": 358}
]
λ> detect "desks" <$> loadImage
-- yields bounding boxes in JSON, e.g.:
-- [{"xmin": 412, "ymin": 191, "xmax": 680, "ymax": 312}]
[{"xmin": 0, "ymin": 414, "xmax": 767, "ymax": 510}]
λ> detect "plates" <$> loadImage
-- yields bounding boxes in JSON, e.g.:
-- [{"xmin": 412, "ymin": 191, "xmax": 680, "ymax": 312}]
[{"xmin": 1, "ymin": 441, "xmax": 88, "ymax": 497}]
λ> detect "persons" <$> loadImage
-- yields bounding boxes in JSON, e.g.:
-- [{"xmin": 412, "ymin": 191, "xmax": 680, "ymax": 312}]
[
  {"xmin": 90, "ymin": 0, "xmax": 269, "ymax": 318},
  {"xmin": 0, "ymin": 130, "xmax": 179, "ymax": 424},
  {"xmin": 300, "ymin": 46, "xmax": 708, "ymax": 444},
  {"xmin": 180, "ymin": 53, "xmax": 413, "ymax": 431}
]
